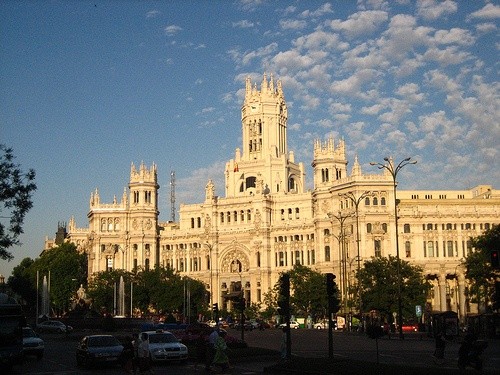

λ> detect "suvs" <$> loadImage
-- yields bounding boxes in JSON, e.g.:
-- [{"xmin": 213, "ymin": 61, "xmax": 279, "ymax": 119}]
[{"xmin": 20, "ymin": 326, "xmax": 45, "ymax": 359}]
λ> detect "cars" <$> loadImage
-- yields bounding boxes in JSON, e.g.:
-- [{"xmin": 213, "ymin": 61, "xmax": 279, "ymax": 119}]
[
  {"xmin": 37, "ymin": 320, "xmax": 74, "ymax": 335},
  {"xmin": 75, "ymin": 334, "xmax": 124, "ymax": 370},
  {"xmin": 131, "ymin": 329, "xmax": 188, "ymax": 367},
  {"xmin": 140, "ymin": 313, "xmax": 419, "ymax": 331}
]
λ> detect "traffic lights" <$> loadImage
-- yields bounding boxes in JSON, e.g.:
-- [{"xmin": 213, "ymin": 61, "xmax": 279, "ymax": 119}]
[
  {"xmin": 490, "ymin": 250, "xmax": 500, "ymax": 266},
  {"xmin": 280, "ymin": 274, "xmax": 290, "ymax": 297},
  {"xmin": 213, "ymin": 303, "xmax": 218, "ymax": 313},
  {"xmin": 240, "ymin": 297, "xmax": 245, "ymax": 311},
  {"xmin": 326, "ymin": 273, "xmax": 337, "ymax": 295}
]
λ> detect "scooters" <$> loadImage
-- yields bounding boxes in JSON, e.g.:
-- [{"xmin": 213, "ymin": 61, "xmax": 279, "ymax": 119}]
[{"xmin": 457, "ymin": 337, "xmax": 489, "ymax": 372}]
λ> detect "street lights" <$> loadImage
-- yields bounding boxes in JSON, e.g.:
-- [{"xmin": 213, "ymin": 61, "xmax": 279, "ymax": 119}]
[
  {"xmin": 325, "ymin": 211, "xmax": 352, "ymax": 322},
  {"xmin": 204, "ymin": 240, "xmax": 223, "ymax": 320},
  {"xmin": 369, "ymin": 156, "xmax": 418, "ymax": 338},
  {"xmin": 332, "ymin": 232, "xmax": 348, "ymax": 314},
  {"xmin": 336, "ymin": 191, "xmax": 375, "ymax": 335}
]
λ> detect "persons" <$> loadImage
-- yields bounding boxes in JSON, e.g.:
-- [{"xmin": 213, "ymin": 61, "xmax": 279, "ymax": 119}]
[
  {"xmin": 206, "ymin": 323, "xmax": 234, "ymax": 371},
  {"xmin": 434, "ymin": 331, "xmax": 450, "ymax": 360},
  {"xmin": 383, "ymin": 323, "xmax": 389, "ymax": 336},
  {"xmin": 457, "ymin": 335, "xmax": 484, "ymax": 375},
  {"xmin": 221, "ymin": 313, "xmax": 233, "ymax": 323},
  {"xmin": 199, "ymin": 313, "xmax": 205, "ymax": 323},
  {"xmin": 259, "ymin": 320, "xmax": 265, "ymax": 331},
  {"xmin": 121, "ymin": 333, "xmax": 150, "ymax": 375}
]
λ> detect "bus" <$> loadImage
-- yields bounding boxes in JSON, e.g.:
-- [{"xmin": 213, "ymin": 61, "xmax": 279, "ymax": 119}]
[{"xmin": 0, "ymin": 302, "xmax": 30, "ymax": 373}]
